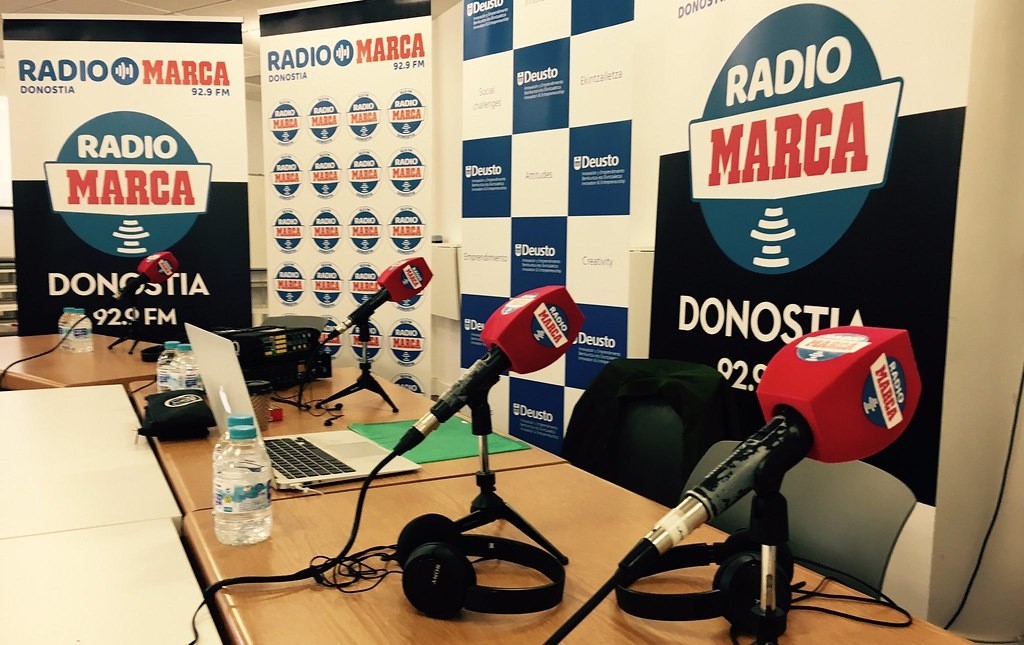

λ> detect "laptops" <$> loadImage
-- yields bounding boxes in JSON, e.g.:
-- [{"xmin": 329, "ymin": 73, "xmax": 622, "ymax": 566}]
[{"xmin": 185, "ymin": 323, "xmax": 422, "ymax": 489}]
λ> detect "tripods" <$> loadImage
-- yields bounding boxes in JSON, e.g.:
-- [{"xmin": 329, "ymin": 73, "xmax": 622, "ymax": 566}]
[
  {"xmin": 454, "ymin": 381, "xmax": 570, "ymax": 566},
  {"xmin": 109, "ymin": 296, "xmax": 152, "ymax": 354},
  {"xmin": 315, "ymin": 306, "xmax": 398, "ymax": 413}
]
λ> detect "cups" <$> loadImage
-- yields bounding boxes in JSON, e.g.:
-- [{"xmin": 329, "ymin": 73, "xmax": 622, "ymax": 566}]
[{"xmin": 245, "ymin": 380, "xmax": 271, "ymax": 431}]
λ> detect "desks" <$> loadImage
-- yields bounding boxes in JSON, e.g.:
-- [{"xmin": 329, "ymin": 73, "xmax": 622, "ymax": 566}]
[{"xmin": 0, "ymin": 333, "xmax": 982, "ymax": 645}]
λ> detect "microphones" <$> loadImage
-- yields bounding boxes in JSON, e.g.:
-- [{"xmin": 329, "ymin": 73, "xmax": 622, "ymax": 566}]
[
  {"xmin": 621, "ymin": 325, "xmax": 923, "ymax": 579},
  {"xmin": 113, "ymin": 252, "xmax": 178, "ymax": 300},
  {"xmin": 326, "ymin": 256, "xmax": 430, "ymax": 340},
  {"xmin": 394, "ymin": 286, "xmax": 585, "ymax": 455}
]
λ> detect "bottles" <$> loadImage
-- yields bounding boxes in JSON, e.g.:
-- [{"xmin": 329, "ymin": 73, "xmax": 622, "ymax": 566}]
[
  {"xmin": 211, "ymin": 414, "xmax": 272, "ymax": 547},
  {"xmin": 57, "ymin": 307, "xmax": 93, "ymax": 354},
  {"xmin": 169, "ymin": 344, "xmax": 204, "ymax": 392},
  {"xmin": 156, "ymin": 341, "xmax": 180, "ymax": 392}
]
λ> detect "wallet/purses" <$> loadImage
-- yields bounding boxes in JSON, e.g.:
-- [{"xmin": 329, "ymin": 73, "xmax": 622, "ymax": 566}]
[{"xmin": 138, "ymin": 390, "xmax": 216, "ymax": 438}]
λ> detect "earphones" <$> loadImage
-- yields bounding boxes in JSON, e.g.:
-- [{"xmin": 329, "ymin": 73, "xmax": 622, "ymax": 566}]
[
  {"xmin": 324, "ymin": 418, "xmax": 337, "ymax": 426},
  {"xmin": 326, "ymin": 403, "xmax": 342, "ymax": 411}
]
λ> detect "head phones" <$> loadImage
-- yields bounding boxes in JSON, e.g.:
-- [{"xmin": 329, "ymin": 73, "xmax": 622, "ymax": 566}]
[
  {"xmin": 617, "ymin": 526, "xmax": 794, "ymax": 622},
  {"xmin": 395, "ymin": 514, "xmax": 566, "ymax": 619}
]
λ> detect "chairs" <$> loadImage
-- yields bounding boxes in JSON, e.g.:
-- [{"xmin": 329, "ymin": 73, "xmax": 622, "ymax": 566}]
[
  {"xmin": 674, "ymin": 431, "xmax": 936, "ymax": 619},
  {"xmin": 555, "ymin": 357, "xmax": 735, "ymax": 504}
]
